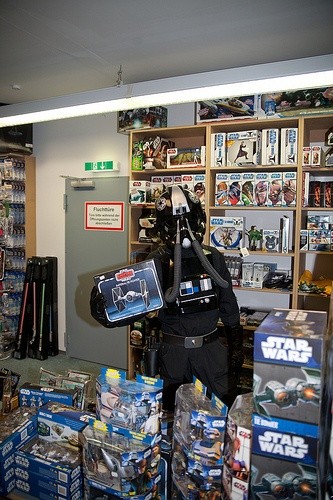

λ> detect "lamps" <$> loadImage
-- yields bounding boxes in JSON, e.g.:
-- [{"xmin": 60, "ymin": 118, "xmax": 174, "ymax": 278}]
[{"xmin": 0, "ymin": 53, "xmax": 333, "ymax": 127}]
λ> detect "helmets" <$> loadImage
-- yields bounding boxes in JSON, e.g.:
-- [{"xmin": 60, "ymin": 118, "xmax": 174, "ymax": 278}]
[{"xmin": 155, "ymin": 185, "xmax": 207, "ymax": 248}]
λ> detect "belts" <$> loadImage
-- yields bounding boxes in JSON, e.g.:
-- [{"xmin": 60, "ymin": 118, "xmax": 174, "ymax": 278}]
[{"xmin": 162, "ymin": 331, "xmax": 218, "ymax": 348}]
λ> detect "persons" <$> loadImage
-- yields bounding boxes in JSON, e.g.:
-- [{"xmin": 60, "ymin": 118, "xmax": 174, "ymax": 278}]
[{"xmin": 90, "ymin": 185, "xmax": 245, "ymax": 408}]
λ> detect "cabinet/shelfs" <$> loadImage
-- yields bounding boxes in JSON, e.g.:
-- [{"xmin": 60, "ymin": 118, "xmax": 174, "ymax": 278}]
[
  {"xmin": 128, "ymin": 322, "xmax": 258, "ymax": 389},
  {"xmin": 127, "ymin": 115, "xmax": 333, "ymax": 335}
]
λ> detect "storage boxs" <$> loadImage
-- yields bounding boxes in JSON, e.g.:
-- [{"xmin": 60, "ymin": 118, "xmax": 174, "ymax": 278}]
[
  {"xmin": 302, "ymin": 172, "xmax": 333, "ymax": 207},
  {"xmin": 0, "ymin": 368, "xmax": 252, "ymax": 500},
  {"xmin": 196, "ymin": 87, "xmax": 333, "ymax": 124},
  {"xmin": 167, "ymin": 145, "xmax": 206, "ymax": 169},
  {"xmin": 128, "ymin": 174, "xmax": 205, "ymax": 205},
  {"xmin": 132, "ymin": 156, "xmax": 143, "ymax": 171},
  {"xmin": 210, "ymin": 216, "xmax": 282, "ymax": 253},
  {"xmin": 300, "ymin": 215, "xmax": 333, "ymax": 252},
  {"xmin": 303, "ymin": 146, "xmax": 326, "ymax": 167},
  {"xmin": 215, "ymin": 172, "xmax": 297, "ymax": 208},
  {"xmin": 248, "ymin": 308, "xmax": 333, "ymax": 500},
  {"xmin": 92, "ymin": 258, "xmax": 167, "ymax": 323},
  {"xmin": 242, "ymin": 262, "xmax": 292, "ymax": 289},
  {"xmin": 117, "ymin": 106, "xmax": 167, "ymax": 135},
  {"xmin": 210, "ymin": 128, "xmax": 298, "ymax": 167}
]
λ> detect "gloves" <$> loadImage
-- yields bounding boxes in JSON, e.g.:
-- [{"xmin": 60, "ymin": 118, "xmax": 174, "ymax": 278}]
[
  {"xmin": 89, "ymin": 285, "xmax": 106, "ymax": 326},
  {"xmin": 226, "ymin": 326, "xmax": 244, "ymax": 372}
]
